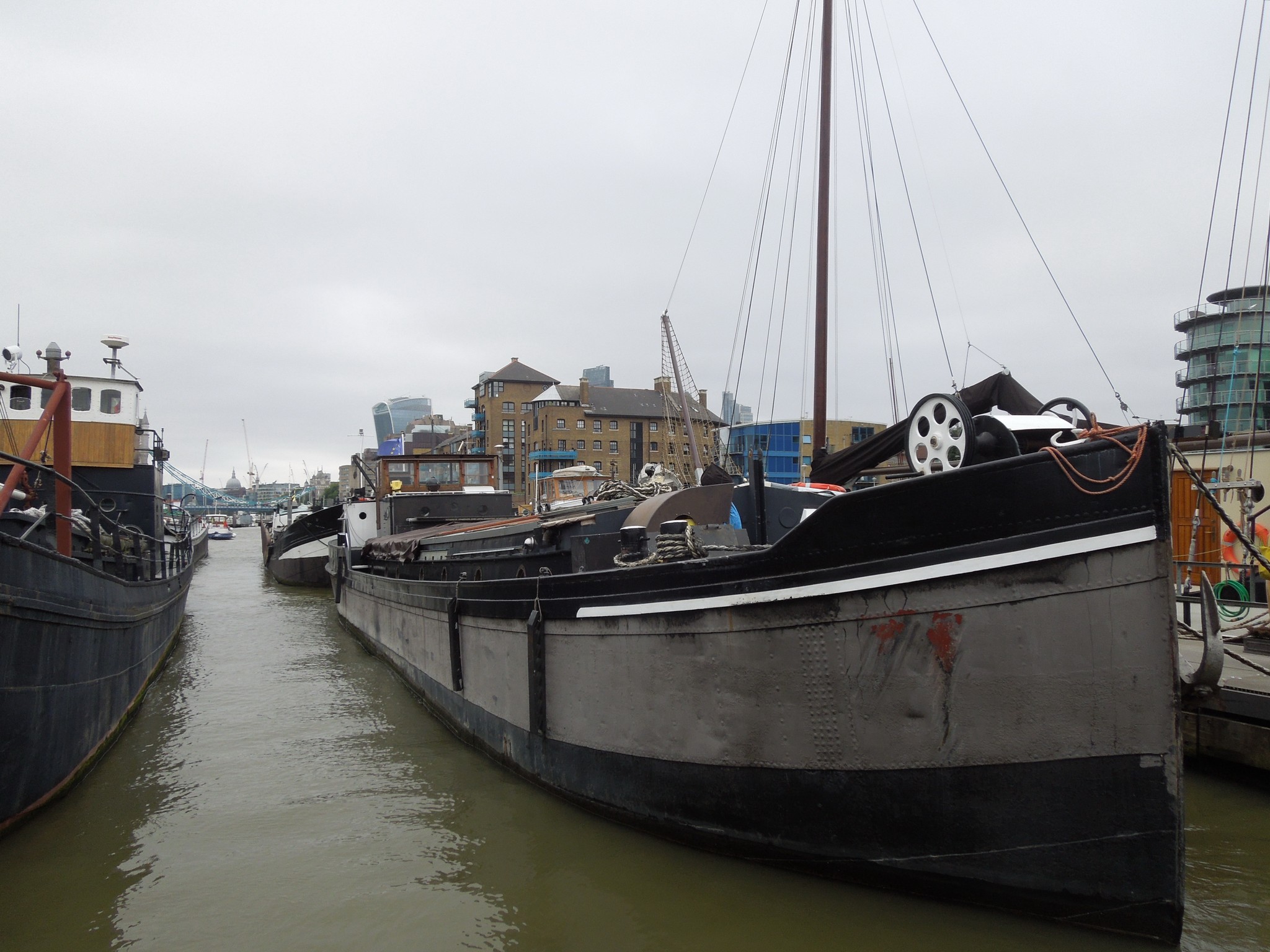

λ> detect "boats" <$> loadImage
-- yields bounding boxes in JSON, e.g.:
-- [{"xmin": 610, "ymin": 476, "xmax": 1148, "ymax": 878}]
[
  {"xmin": 1, "ymin": 300, "xmax": 236, "ymax": 841},
  {"xmin": 259, "ymin": 490, "xmax": 349, "ymax": 589}
]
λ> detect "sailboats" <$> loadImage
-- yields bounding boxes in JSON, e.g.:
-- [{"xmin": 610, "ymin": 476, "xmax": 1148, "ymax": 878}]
[{"xmin": 326, "ymin": 2, "xmax": 1190, "ymax": 951}]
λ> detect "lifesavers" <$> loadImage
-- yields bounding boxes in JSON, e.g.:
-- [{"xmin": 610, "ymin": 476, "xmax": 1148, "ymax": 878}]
[
  {"xmin": 1221, "ymin": 521, "xmax": 1270, "ymax": 577},
  {"xmin": 349, "ymin": 498, "xmax": 372, "ymax": 501},
  {"xmin": 787, "ymin": 481, "xmax": 846, "ymax": 493}
]
